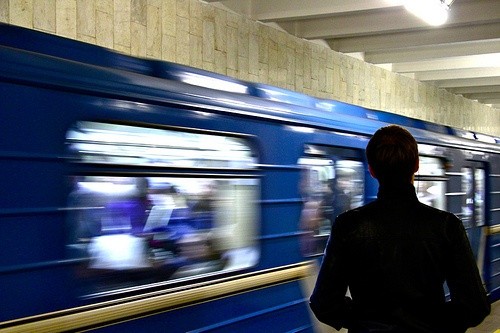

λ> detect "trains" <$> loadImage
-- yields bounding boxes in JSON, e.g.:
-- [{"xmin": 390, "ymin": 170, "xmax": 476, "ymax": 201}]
[{"xmin": 0, "ymin": 23, "xmax": 500, "ymax": 333}]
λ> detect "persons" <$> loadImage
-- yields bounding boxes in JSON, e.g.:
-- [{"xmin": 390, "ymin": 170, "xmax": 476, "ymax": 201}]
[
  {"xmin": 75, "ymin": 168, "xmax": 487, "ymax": 284},
  {"xmin": 310, "ymin": 126, "xmax": 491, "ymax": 333}
]
